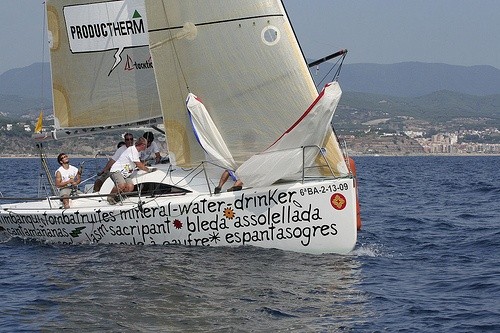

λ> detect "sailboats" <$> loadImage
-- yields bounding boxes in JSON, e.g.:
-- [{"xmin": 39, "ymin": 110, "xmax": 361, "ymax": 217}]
[{"xmin": 0, "ymin": 1, "xmax": 358, "ymax": 256}]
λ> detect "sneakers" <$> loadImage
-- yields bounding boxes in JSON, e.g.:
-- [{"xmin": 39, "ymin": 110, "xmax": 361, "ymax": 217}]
[{"xmin": 107, "ymin": 197, "xmax": 117, "ymax": 205}]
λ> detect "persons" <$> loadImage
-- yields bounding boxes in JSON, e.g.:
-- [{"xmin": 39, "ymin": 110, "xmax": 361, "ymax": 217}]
[
  {"xmin": 55, "ymin": 153, "xmax": 82, "ymax": 209},
  {"xmin": 94, "ymin": 132, "xmax": 161, "ymax": 206},
  {"xmin": 214, "ymin": 172, "xmax": 243, "ymax": 193}
]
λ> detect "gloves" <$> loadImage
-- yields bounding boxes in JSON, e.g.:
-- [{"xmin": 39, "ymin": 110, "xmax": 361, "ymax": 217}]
[{"xmin": 147, "ymin": 167, "xmax": 157, "ymax": 173}]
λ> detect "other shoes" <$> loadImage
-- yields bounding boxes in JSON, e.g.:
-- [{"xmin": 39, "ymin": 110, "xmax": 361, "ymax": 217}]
[
  {"xmin": 214, "ymin": 187, "xmax": 221, "ymax": 194},
  {"xmin": 73, "ymin": 192, "xmax": 79, "ymax": 197},
  {"xmin": 63, "ymin": 198, "xmax": 70, "ymax": 209},
  {"xmin": 227, "ymin": 186, "xmax": 242, "ymax": 192}
]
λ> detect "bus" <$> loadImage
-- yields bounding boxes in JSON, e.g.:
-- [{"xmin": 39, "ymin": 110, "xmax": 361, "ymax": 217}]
[
  {"xmin": 6, "ymin": 124, "xmax": 31, "ymax": 132},
  {"xmin": 42, "ymin": 126, "xmax": 52, "ymax": 132}
]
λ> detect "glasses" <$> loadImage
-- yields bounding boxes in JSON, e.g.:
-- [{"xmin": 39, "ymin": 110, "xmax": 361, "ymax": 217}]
[
  {"xmin": 139, "ymin": 144, "xmax": 146, "ymax": 147},
  {"xmin": 61, "ymin": 156, "xmax": 68, "ymax": 159},
  {"xmin": 125, "ymin": 138, "xmax": 133, "ymax": 141}
]
